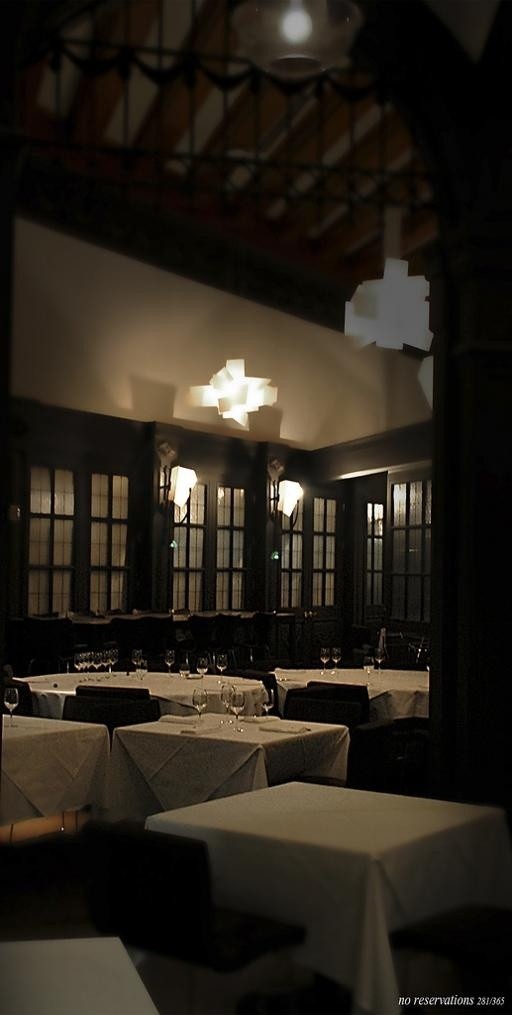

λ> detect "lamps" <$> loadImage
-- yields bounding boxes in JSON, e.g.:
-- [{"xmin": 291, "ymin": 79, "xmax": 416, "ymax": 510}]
[
  {"xmin": 156, "ymin": 439, "xmax": 198, "ymax": 527},
  {"xmin": 268, "ymin": 458, "xmax": 305, "ymax": 536}
]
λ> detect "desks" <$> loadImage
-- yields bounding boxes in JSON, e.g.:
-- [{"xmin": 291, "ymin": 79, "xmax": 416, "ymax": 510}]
[
  {"xmin": 4, "ymin": 608, "xmax": 299, "ymax": 664},
  {"xmin": 0, "ymin": 668, "xmax": 511, "ymax": 1015}
]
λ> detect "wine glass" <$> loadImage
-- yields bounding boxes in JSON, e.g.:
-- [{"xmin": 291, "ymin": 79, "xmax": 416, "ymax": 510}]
[
  {"xmin": 163, "ymin": 649, "xmax": 230, "ymax": 688},
  {"xmin": 189, "ymin": 686, "xmax": 275, "ymax": 733},
  {"xmin": 320, "ymin": 646, "xmax": 385, "ymax": 688},
  {"xmin": 71, "ymin": 647, "xmax": 148, "ymax": 686},
  {"xmin": 1, "ymin": 688, "xmax": 21, "ymax": 728}
]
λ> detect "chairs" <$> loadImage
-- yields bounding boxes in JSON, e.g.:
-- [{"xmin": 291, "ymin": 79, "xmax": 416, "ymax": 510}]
[{"xmin": 21, "ymin": 614, "xmax": 272, "ymax": 674}]
